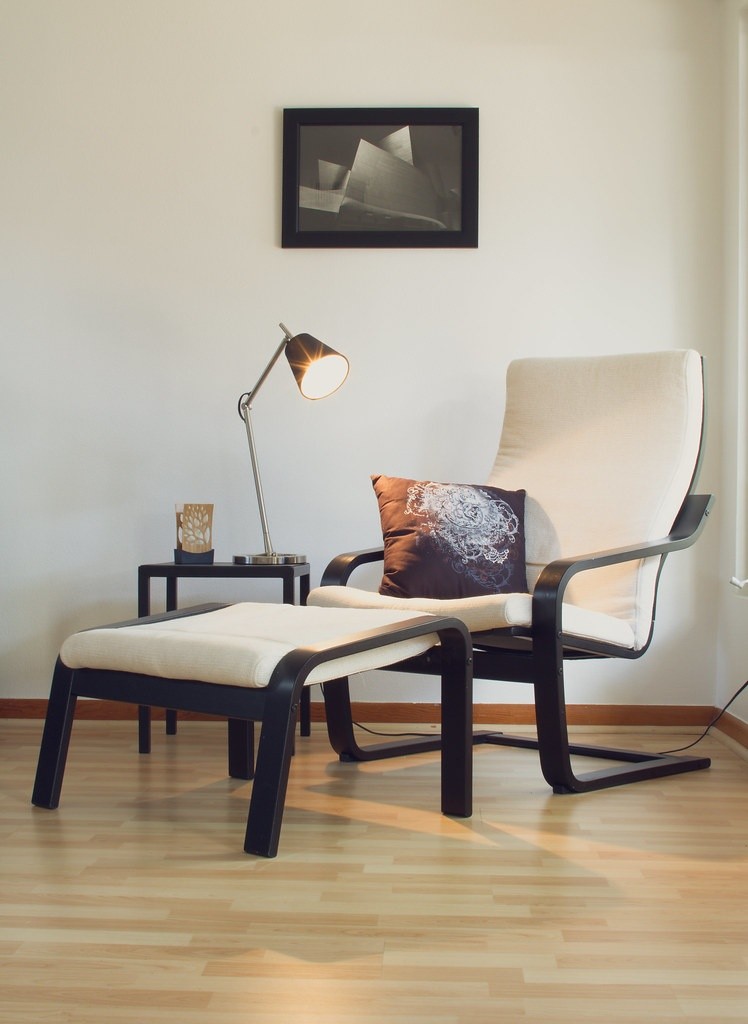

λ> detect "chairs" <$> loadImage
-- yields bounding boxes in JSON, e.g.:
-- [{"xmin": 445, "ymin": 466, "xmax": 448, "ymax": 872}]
[{"xmin": 30, "ymin": 348, "xmax": 715, "ymax": 860}]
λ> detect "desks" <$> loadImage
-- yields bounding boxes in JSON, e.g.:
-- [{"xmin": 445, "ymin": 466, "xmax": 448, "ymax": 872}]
[{"xmin": 136, "ymin": 561, "xmax": 311, "ymax": 755}]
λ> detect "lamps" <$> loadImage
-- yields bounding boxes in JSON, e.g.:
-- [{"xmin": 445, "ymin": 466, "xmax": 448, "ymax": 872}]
[{"xmin": 236, "ymin": 321, "xmax": 351, "ymax": 563}]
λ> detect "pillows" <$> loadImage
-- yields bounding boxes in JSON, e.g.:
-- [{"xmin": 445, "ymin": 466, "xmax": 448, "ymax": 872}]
[{"xmin": 369, "ymin": 473, "xmax": 532, "ymax": 599}]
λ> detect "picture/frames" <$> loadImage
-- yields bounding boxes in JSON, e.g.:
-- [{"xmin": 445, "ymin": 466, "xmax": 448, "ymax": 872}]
[{"xmin": 281, "ymin": 107, "xmax": 481, "ymax": 250}]
